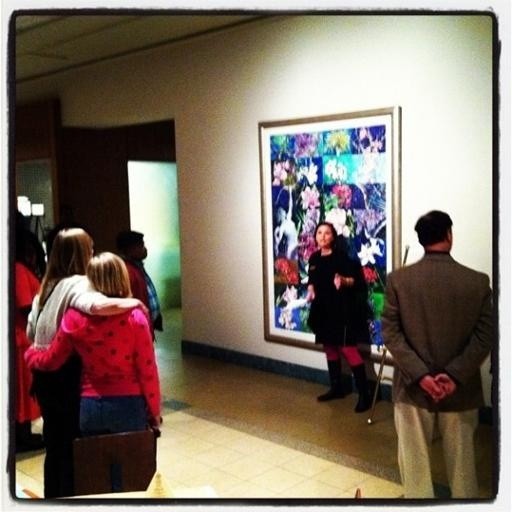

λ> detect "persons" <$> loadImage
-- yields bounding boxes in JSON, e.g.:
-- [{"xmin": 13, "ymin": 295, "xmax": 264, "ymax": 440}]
[
  {"xmin": 23, "ymin": 252, "xmax": 162, "ymax": 436},
  {"xmin": 307, "ymin": 223, "xmax": 373, "ymax": 413},
  {"xmin": 14, "ymin": 251, "xmax": 43, "ymax": 453},
  {"xmin": 115, "ymin": 231, "xmax": 163, "ymax": 343},
  {"xmin": 26, "ymin": 227, "xmax": 101, "ymax": 499},
  {"xmin": 381, "ymin": 210, "xmax": 495, "ymax": 498}
]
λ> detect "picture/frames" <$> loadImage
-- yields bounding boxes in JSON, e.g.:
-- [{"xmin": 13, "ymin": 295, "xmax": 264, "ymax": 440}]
[{"xmin": 258, "ymin": 105, "xmax": 403, "ymax": 368}]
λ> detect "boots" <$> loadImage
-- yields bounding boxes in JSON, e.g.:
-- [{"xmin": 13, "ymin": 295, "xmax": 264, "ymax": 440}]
[
  {"xmin": 318, "ymin": 357, "xmax": 343, "ymax": 402},
  {"xmin": 351, "ymin": 362, "xmax": 374, "ymax": 412}
]
